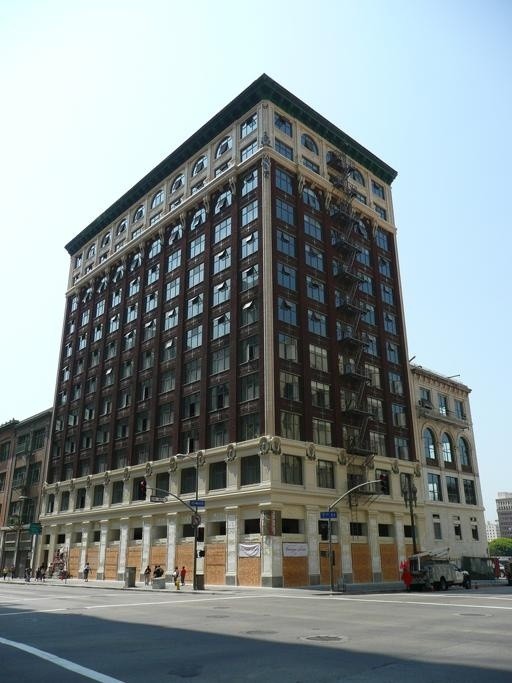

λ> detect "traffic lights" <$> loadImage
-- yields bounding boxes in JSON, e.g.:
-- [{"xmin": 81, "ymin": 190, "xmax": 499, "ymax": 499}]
[
  {"xmin": 137, "ymin": 479, "xmax": 147, "ymax": 500},
  {"xmin": 380, "ymin": 473, "xmax": 389, "ymax": 495}
]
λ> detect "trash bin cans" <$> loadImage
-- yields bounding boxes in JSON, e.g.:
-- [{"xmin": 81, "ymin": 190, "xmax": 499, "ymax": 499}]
[{"xmin": 124, "ymin": 566, "xmax": 136, "ymax": 587}]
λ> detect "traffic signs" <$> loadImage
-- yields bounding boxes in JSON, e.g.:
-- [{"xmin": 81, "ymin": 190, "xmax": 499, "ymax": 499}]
[
  {"xmin": 190, "ymin": 499, "xmax": 205, "ymax": 506},
  {"xmin": 149, "ymin": 495, "xmax": 168, "ymax": 503},
  {"xmin": 319, "ymin": 510, "xmax": 338, "ymax": 518}
]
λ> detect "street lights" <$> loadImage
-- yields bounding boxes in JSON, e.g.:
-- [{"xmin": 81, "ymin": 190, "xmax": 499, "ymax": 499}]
[
  {"xmin": 18, "ymin": 494, "xmax": 34, "ymax": 575},
  {"xmin": 176, "ymin": 451, "xmax": 202, "ymax": 588},
  {"xmin": 402, "ymin": 483, "xmax": 418, "ymax": 552}
]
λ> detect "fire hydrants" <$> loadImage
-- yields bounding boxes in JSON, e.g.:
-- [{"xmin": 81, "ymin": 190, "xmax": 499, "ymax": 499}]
[{"xmin": 175, "ymin": 578, "xmax": 181, "ymax": 589}]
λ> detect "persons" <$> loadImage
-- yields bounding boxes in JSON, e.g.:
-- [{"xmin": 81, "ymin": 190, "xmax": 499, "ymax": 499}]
[
  {"xmin": 144, "ymin": 565, "xmax": 164, "ymax": 586},
  {"xmin": 83, "ymin": 562, "xmax": 92, "ymax": 582},
  {"xmin": 0, "ymin": 563, "xmax": 46, "ymax": 583},
  {"xmin": 181, "ymin": 566, "xmax": 186, "ymax": 586},
  {"xmin": 173, "ymin": 567, "xmax": 179, "ymax": 586}
]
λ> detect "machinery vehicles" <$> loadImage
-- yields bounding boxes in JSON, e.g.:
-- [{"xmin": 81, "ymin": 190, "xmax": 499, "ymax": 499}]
[{"xmin": 402, "ymin": 547, "xmax": 471, "ymax": 590}]
